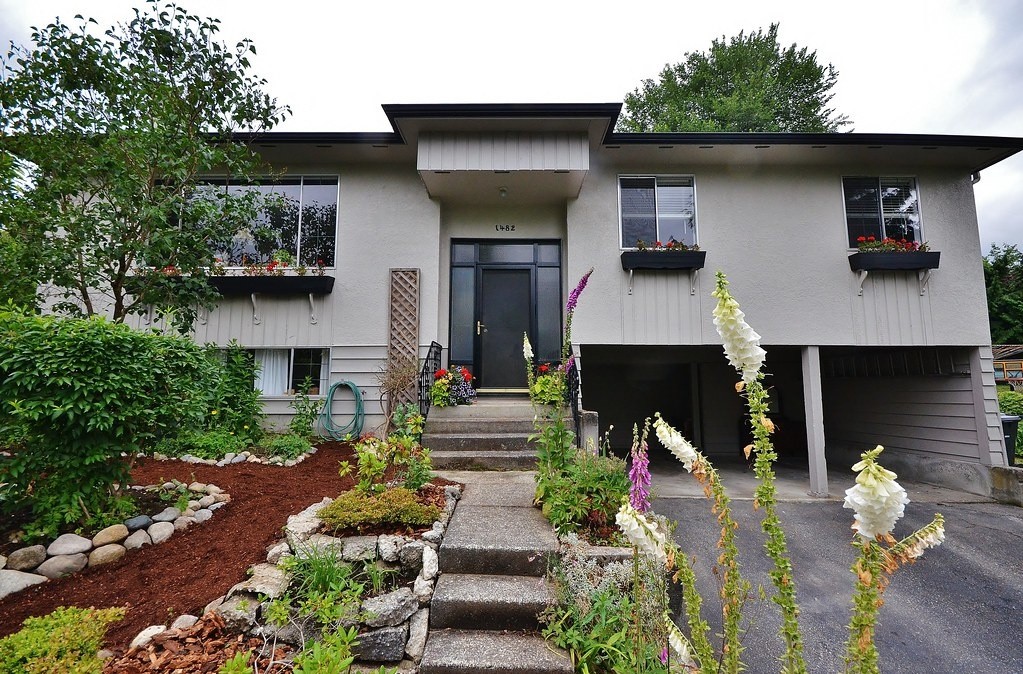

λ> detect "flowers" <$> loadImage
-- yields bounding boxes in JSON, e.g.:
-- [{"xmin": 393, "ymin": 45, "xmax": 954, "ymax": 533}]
[
  {"xmin": 531, "ymin": 361, "xmax": 565, "ymax": 405},
  {"xmin": 857, "ymin": 233, "xmax": 931, "ymax": 252},
  {"xmin": 429, "ymin": 364, "xmax": 477, "ymax": 409},
  {"xmin": 153, "ymin": 256, "xmax": 326, "ymax": 276},
  {"xmin": 636, "ymin": 239, "xmax": 700, "ymax": 251}
]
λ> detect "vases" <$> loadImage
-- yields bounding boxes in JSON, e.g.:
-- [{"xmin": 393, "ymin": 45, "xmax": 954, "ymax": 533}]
[
  {"xmin": 848, "ymin": 252, "xmax": 941, "ymax": 272},
  {"xmin": 620, "ymin": 251, "xmax": 707, "ymax": 271},
  {"xmin": 124, "ymin": 276, "xmax": 336, "ymax": 294}
]
